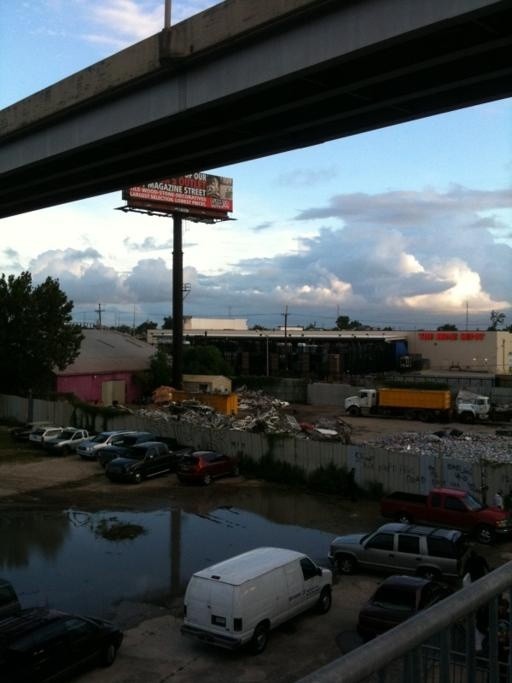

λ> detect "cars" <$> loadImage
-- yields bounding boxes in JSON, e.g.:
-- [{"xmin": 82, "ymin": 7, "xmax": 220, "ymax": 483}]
[
  {"xmin": 12, "ymin": 419, "xmax": 241, "ymax": 486},
  {"xmin": 169, "ymin": 399, "xmax": 216, "ymax": 418},
  {"xmin": 358, "ymin": 573, "xmax": 440, "ymax": 642}
]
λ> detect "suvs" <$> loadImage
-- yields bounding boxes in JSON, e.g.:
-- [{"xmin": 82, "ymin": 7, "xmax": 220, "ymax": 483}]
[{"xmin": 327, "ymin": 521, "xmax": 472, "ymax": 581}]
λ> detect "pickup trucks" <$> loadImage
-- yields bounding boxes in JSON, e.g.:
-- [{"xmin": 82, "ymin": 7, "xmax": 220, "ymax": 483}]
[{"xmin": 378, "ymin": 486, "xmax": 511, "ymax": 544}]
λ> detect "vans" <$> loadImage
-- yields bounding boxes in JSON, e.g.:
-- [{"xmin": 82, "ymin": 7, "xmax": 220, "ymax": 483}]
[
  {"xmin": 180, "ymin": 543, "xmax": 335, "ymax": 653},
  {"xmin": 0, "ymin": 607, "xmax": 123, "ymax": 682}
]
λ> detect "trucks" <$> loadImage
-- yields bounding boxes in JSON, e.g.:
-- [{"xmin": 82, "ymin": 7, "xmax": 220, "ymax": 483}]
[
  {"xmin": 344, "ymin": 386, "xmax": 452, "ymax": 422},
  {"xmin": 456, "ymin": 394, "xmax": 511, "ymax": 426}
]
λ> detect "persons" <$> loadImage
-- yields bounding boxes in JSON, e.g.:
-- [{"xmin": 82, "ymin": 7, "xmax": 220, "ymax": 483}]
[
  {"xmin": 113, "ymin": 399, "xmax": 125, "ymax": 410},
  {"xmin": 493, "ymin": 489, "xmax": 506, "ymax": 509}
]
